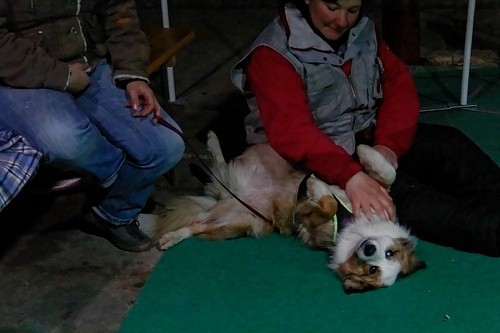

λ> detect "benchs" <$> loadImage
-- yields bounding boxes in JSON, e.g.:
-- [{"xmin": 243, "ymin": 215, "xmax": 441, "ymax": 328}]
[{"xmin": 0, "ymin": 0, "xmax": 196, "ymax": 216}]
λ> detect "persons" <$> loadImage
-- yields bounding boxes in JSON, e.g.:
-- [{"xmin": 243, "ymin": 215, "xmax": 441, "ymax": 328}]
[
  {"xmin": 0, "ymin": 0, "xmax": 185, "ymax": 251},
  {"xmin": 230, "ymin": 0, "xmax": 500, "ymax": 257}
]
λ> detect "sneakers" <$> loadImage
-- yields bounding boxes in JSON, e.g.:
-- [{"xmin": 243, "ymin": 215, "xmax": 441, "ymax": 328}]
[
  {"xmin": 150, "ymin": 171, "xmax": 174, "ymax": 205},
  {"xmin": 85, "ymin": 212, "xmax": 151, "ymax": 252}
]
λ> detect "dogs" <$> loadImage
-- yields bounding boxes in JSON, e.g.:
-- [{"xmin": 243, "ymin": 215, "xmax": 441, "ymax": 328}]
[{"xmin": 158, "ymin": 130, "xmax": 426, "ymax": 294}]
